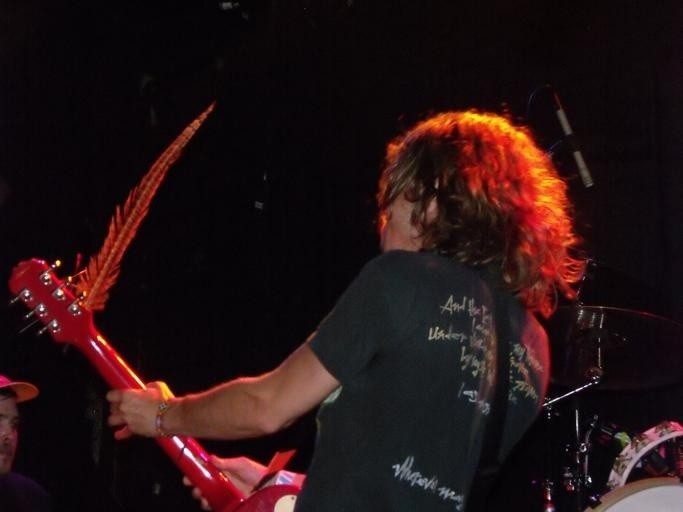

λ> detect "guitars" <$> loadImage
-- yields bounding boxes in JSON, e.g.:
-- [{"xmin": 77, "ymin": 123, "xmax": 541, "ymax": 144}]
[{"xmin": 9, "ymin": 257, "xmax": 302, "ymax": 512}]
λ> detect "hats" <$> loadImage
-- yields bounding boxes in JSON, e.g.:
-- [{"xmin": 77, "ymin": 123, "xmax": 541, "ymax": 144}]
[{"xmin": 0, "ymin": 373, "xmax": 41, "ymax": 403}]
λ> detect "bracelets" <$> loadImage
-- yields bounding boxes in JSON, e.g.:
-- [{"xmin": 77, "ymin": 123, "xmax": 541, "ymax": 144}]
[{"xmin": 153, "ymin": 394, "xmax": 173, "ymax": 441}]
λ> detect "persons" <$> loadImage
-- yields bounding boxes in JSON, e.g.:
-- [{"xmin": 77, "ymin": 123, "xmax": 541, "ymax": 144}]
[
  {"xmin": 0, "ymin": 374, "xmax": 51, "ymax": 511},
  {"xmin": 96, "ymin": 106, "xmax": 592, "ymax": 511}
]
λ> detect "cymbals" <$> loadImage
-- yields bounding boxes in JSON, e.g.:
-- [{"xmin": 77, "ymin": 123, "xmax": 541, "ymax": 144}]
[{"xmin": 551, "ymin": 306, "xmax": 683, "ymax": 389}]
